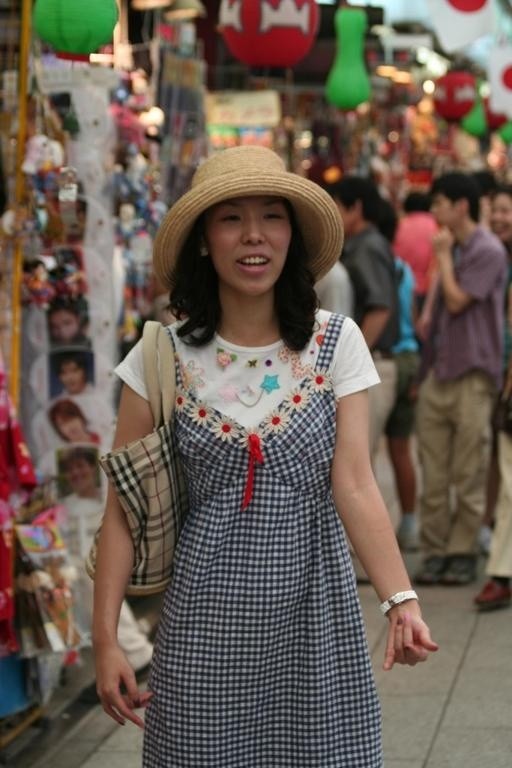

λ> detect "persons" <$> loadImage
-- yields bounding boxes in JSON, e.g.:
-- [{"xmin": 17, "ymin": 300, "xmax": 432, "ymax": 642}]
[
  {"xmin": 89, "ymin": 143, "xmax": 440, "ymax": 768},
  {"xmin": 314, "ymin": 168, "xmax": 512, "ymax": 616},
  {"xmin": 43, "ymin": 297, "xmax": 100, "ymax": 499}
]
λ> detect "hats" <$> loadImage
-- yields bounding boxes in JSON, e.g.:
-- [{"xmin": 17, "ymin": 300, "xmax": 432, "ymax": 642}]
[{"xmin": 152, "ymin": 144, "xmax": 346, "ymax": 290}]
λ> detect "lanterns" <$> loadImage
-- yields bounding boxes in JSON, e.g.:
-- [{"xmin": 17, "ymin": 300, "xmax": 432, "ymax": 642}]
[
  {"xmin": 33, "ymin": 0, "xmax": 120, "ymax": 63},
  {"xmin": 215, "ymin": 1, "xmax": 320, "ymax": 69}
]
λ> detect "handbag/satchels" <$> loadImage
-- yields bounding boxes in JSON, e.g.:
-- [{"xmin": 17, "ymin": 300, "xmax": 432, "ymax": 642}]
[{"xmin": 81, "ymin": 420, "xmax": 187, "ymax": 601}]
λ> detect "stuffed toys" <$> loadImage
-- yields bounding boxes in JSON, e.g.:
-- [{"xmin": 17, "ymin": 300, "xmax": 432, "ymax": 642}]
[
  {"xmin": 1, "ymin": 62, "xmax": 168, "ymax": 344},
  {"xmin": 0, "ymin": 61, "xmax": 167, "ymax": 342}
]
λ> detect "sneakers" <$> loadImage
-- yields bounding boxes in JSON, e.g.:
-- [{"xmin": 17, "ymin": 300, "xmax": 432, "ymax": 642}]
[{"xmin": 395, "ymin": 513, "xmax": 512, "ymax": 609}]
